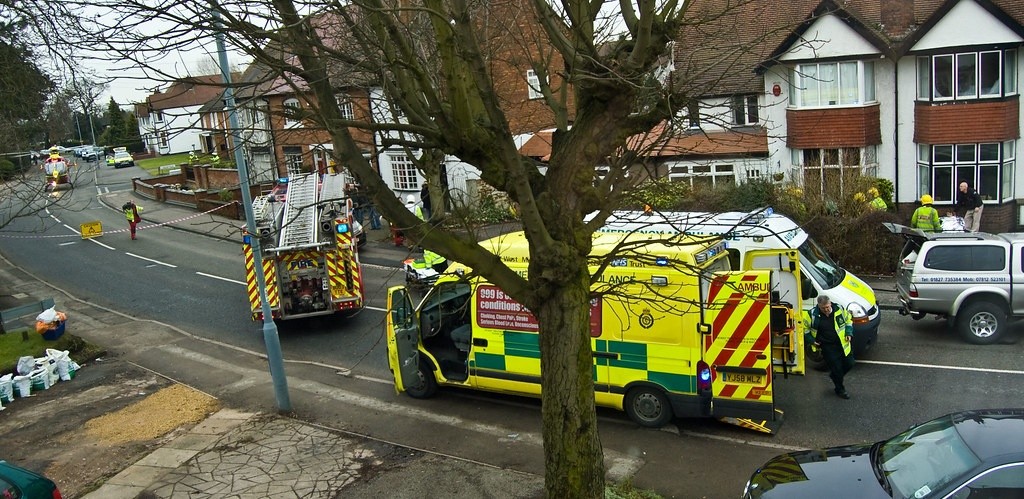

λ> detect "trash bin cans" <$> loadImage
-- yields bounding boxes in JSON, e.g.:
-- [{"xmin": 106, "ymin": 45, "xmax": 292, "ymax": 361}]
[{"xmin": 35, "ymin": 308, "xmax": 68, "ymax": 341}]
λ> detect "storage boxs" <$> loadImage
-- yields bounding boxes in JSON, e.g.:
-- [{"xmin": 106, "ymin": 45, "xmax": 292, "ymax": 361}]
[{"xmin": 41, "ymin": 321, "xmax": 66, "ymax": 341}]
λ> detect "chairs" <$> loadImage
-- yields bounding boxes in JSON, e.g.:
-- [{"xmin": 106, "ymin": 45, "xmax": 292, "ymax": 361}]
[{"xmin": 451, "ymin": 324, "xmax": 471, "ymax": 353}]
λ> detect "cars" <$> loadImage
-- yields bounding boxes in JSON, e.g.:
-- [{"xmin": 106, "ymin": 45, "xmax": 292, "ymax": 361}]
[
  {"xmin": 742, "ymin": 408, "xmax": 1024, "ymax": 499},
  {"xmin": 113, "ymin": 146, "xmax": 134, "ymax": 169},
  {"xmin": 58, "ymin": 144, "xmax": 116, "ymax": 166}
]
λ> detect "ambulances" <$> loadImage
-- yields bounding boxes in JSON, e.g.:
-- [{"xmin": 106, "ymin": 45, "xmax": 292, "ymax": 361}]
[
  {"xmin": 582, "ymin": 206, "xmax": 881, "ymax": 373},
  {"xmin": 387, "ymin": 229, "xmax": 807, "ymax": 436}
]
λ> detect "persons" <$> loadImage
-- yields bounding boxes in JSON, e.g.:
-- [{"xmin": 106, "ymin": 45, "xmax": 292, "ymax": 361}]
[
  {"xmin": 189, "ymin": 152, "xmax": 198, "ymax": 164},
  {"xmin": 854, "ymin": 187, "xmax": 888, "ymax": 213},
  {"xmin": 802, "ymin": 295, "xmax": 856, "ymax": 399},
  {"xmin": 352, "ymin": 189, "xmax": 381, "ymax": 230},
  {"xmin": 423, "ymin": 249, "xmax": 448, "ymax": 275},
  {"xmin": 404, "ymin": 194, "xmax": 423, "ymax": 253},
  {"xmin": 421, "ymin": 182, "xmax": 431, "ymax": 223},
  {"xmin": 209, "ymin": 152, "xmax": 221, "ymax": 166},
  {"xmin": 122, "ymin": 200, "xmax": 144, "ymax": 240},
  {"xmin": 911, "ymin": 182, "xmax": 984, "ymax": 233}
]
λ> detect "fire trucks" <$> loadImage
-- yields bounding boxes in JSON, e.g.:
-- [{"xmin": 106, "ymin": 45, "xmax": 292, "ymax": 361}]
[{"xmin": 239, "ymin": 170, "xmax": 365, "ymax": 330}]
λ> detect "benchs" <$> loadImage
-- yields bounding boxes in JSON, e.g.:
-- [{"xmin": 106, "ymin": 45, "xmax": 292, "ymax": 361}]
[{"xmin": 0, "ymin": 297, "xmax": 54, "ymax": 341}]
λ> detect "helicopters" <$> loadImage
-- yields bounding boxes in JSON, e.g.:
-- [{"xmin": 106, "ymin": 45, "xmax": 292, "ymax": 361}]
[{"xmin": 0, "ymin": 144, "xmax": 114, "ymax": 192}]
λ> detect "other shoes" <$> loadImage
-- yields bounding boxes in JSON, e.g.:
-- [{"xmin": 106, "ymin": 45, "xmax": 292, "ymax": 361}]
[{"xmin": 835, "ymin": 388, "xmax": 849, "ymax": 399}]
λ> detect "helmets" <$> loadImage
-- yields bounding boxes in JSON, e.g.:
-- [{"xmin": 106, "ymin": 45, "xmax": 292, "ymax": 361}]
[
  {"xmin": 921, "ymin": 194, "xmax": 932, "ymax": 206},
  {"xmin": 854, "ymin": 192, "xmax": 866, "ymax": 203},
  {"xmin": 867, "ymin": 187, "xmax": 879, "ymax": 198},
  {"xmin": 407, "ymin": 194, "xmax": 416, "ymax": 204}
]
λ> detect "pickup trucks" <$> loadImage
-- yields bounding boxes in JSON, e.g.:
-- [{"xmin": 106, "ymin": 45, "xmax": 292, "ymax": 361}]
[{"xmin": 882, "ymin": 222, "xmax": 1024, "ymax": 346}]
